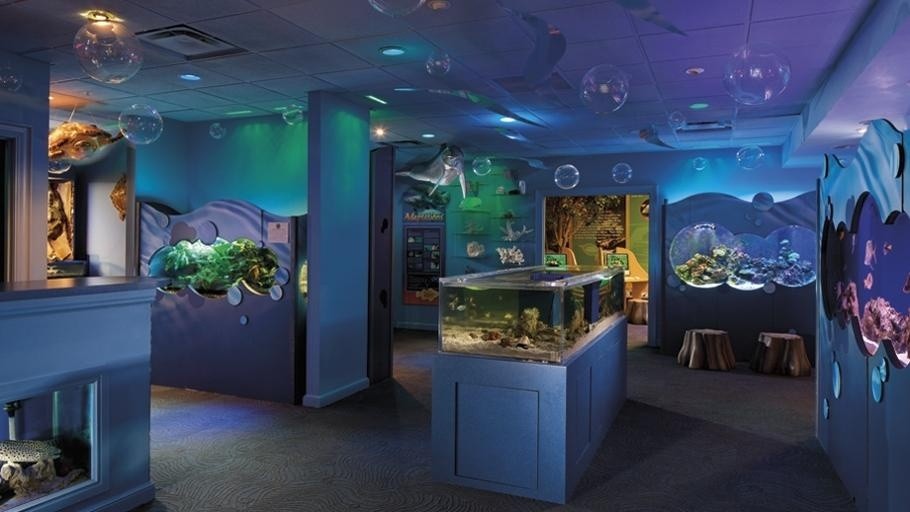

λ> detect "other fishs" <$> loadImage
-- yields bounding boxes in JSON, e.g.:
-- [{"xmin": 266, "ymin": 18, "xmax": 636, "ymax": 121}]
[
  {"xmin": 882, "ymin": 241, "xmax": 893, "ymax": 256},
  {"xmin": 863, "ymin": 272, "xmax": 874, "ymax": 290},
  {"xmin": 615, "ymin": 0, "xmax": 687, "ymax": 36},
  {"xmin": 447, "ymin": 293, "xmax": 548, "ymax": 349},
  {"xmin": 0, "ymin": 439, "xmax": 61, "ymax": 470},
  {"xmin": 864, "ymin": 240, "xmax": 877, "ymax": 271},
  {"xmin": 839, "ymin": 232, "xmax": 845, "ymax": 240}
]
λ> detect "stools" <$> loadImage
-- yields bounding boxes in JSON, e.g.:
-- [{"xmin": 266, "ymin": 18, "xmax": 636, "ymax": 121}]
[
  {"xmin": 627, "ymin": 298, "xmax": 649, "ymax": 325},
  {"xmin": 678, "ymin": 325, "xmax": 740, "ymax": 372},
  {"xmin": 750, "ymin": 330, "xmax": 815, "ymax": 379}
]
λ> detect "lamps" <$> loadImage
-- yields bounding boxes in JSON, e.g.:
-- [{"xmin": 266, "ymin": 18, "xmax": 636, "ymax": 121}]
[{"xmin": 73, "ymin": 9, "xmax": 146, "ymax": 87}]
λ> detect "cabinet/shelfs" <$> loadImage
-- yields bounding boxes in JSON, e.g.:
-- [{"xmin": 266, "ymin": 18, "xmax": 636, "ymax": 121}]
[
  {"xmin": 452, "ymin": 183, "xmax": 532, "ymax": 273},
  {"xmin": 429, "ymin": 312, "xmax": 629, "ymax": 504}
]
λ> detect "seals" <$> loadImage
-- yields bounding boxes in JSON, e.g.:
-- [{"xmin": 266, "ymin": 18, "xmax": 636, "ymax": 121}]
[{"xmin": 394, "ymin": 145, "xmax": 466, "ymax": 199}]
